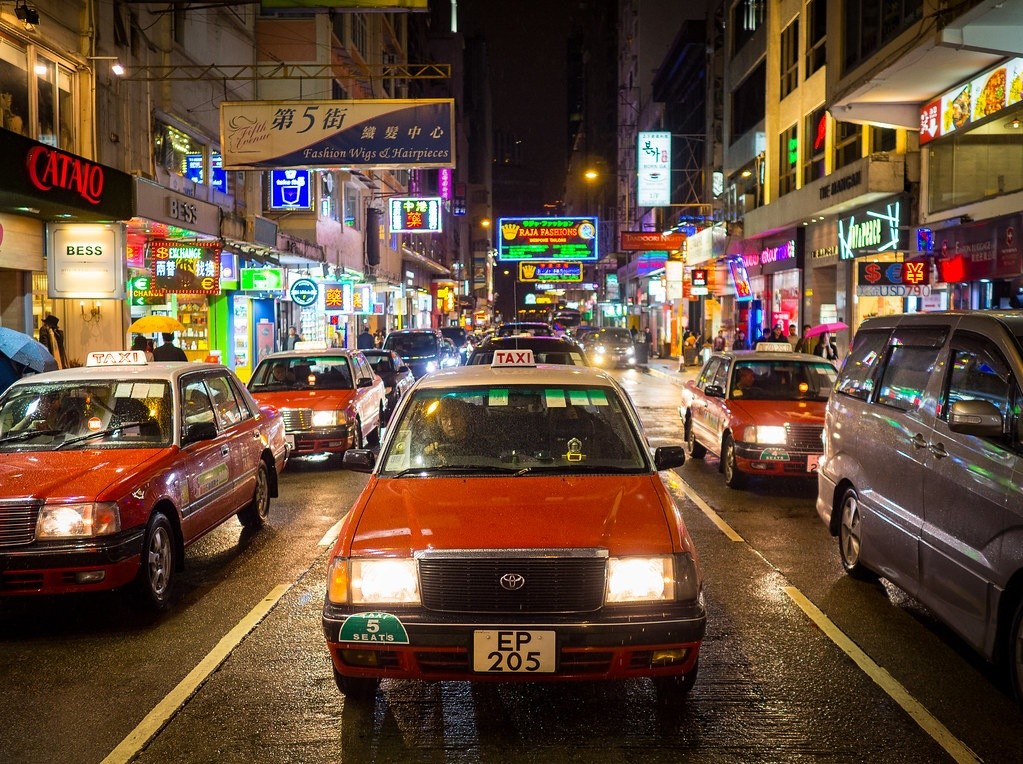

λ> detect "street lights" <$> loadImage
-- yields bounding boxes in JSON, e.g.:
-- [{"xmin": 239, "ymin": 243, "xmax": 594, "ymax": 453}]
[
  {"xmin": 585, "ymin": 172, "xmax": 628, "ymax": 330},
  {"xmin": 469, "ymin": 220, "xmax": 491, "ymax": 331}
]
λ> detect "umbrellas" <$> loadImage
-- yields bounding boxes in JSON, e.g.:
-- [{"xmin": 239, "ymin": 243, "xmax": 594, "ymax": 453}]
[
  {"xmin": 0, "ymin": 327, "xmax": 60, "ymax": 373},
  {"xmin": 805, "ymin": 322, "xmax": 850, "ymax": 339},
  {"xmin": 128, "ymin": 315, "xmax": 185, "ymax": 333}
]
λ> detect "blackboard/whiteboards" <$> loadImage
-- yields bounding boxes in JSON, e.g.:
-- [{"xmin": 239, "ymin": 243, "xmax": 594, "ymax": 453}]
[{"xmin": 684, "ymin": 348, "xmax": 699, "ymax": 365}]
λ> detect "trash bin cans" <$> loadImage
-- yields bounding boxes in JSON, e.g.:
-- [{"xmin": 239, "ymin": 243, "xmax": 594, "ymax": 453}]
[{"xmin": 634, "ymin": 341, "xmax": 648, "ymax": 364}]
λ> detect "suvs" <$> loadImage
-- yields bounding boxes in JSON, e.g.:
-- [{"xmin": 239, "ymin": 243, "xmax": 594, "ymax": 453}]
[{"xmin": 465, "ymin": 334, "xmax": 592, "ymax": 368}]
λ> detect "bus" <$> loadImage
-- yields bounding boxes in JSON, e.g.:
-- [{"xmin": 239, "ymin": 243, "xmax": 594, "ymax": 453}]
[{"xmin": 549, "ymin": 307, "xmax": 584, "ymax": 337}]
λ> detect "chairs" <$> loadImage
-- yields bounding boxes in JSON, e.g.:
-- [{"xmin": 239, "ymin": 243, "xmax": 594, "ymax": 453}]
[
  {"xmin": 293, "ymin": 366, "xmax": 312, "ymax": 384},
  {"xmin": 550, "ymin": 419, "xmax": 602, "ymax": 460},
  {"xmin": 768, "ymin": 370, "xmax": 832, "ymax": 392},
  {"xmin": 101, "ymin": 414, "xmax": 164, "ymax": 442}
]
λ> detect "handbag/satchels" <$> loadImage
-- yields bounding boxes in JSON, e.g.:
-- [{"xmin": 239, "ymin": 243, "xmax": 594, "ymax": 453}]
[{"xmin": 831, "ymin": 355, "xmax": 842, "ymax": 371}]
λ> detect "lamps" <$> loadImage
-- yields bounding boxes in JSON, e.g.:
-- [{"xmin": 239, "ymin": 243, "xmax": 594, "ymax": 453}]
[
  {"xmin": 13, "ymin": 0, "xmax": 41, "ymax": 26},
  {"xmin": 80, "ymin": 299, "xmax": 102, "ymax": 323},
  {"xmin": 1004, "ymin": 110, "xmax": 1023, "ymax": 129},
  {"xmin": 112, "ymin": 58, "xmax": 125, "ymax": 75}
]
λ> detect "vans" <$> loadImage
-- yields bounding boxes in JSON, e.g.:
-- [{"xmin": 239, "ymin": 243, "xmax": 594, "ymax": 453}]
[{"xmin": 817, "ymin": 308, "xmax": 1023, "ymax": 737}]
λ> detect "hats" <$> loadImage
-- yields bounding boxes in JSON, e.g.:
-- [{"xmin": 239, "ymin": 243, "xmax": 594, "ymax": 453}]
[{"xmin": 41, "ymin": 315, "xmax": 59, "ymax": 327}]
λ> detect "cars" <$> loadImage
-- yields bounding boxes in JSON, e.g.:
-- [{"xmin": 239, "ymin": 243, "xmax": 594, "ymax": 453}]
[
  {"xmin": 575, "ymin": 325, "xmax": 649, "ymax": 369},
  {"xmin": 678, "ymin": 350, "xmax": 839, "ymax": 486},
  {"xmin": 358, "ymin": 347, "xmax": 415, "ymax": 428},
  {"xmin": 320, "ymin": 367, "xmax": 707, "ymax": 701},
  {"xmin": 443, "ymin": 337, "xmax": 468, "ymax": 369},
  {"xmin": 0, "ymin": 361, "xmax": 292, "ymax": 612},
  {"xmin": 244, "ymin": 349, "xmax": 388, "ymax": 456},
  {"xmin": 379, "ymin": 328, "xmax": 450, "ymax": 380},
  {"xmin": 438, "ymin": 326, "xmax": 473, "ymax": 364},
  {"xmin": 495, "ymin": 322, "xmax": 556, "ymax": 337}
]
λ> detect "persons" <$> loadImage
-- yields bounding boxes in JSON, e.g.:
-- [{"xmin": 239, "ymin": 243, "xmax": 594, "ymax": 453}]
[
  {"xmin": 331, "ymin": 332, "xmax": 344, "ymax": 348},
  {"xmin": 129, "ymin": 332, "xmax": 189, "ymax": 362},
  {"xmin": 7, "ymin": 391, "xmax": 84, "ymax": 436},
  {"xmin": 713, "ymin": 324, "xmax": 837, "ymax": 365},
  {"xmin": 392, "ymin": 341, "xmax": 407, "ymax": 353},
  {"xmin": 630, "ymin": 325, "xmax": 702, "ymax": 366},
  {"xmin": 356, "ymin": 325, "xmax": 394, "ymax": 348},
  {"xmin": 734, "ymin": 367, "xmax": 755, "ymax": 394},
  {"xmin": 547, "ymin": 402, "xmax": 603, "ymax": 460},
  {"xmin": 282, "ymin": 327, "xmax": 302, "ymax": 351},
  {"xmin": 268, "ymin": 365, "xmax": 293, "ymax": 386},
  {"xmin": 0, "ymin": 351, "xmax": 21, "ymax": 397},
  {"xmin": 410, "ymin": 398, "xmax": 489, "ymax": 458},
  {"xmin": 40, "ymin": 315, "xmax": 67, "ymax": 370}
]
